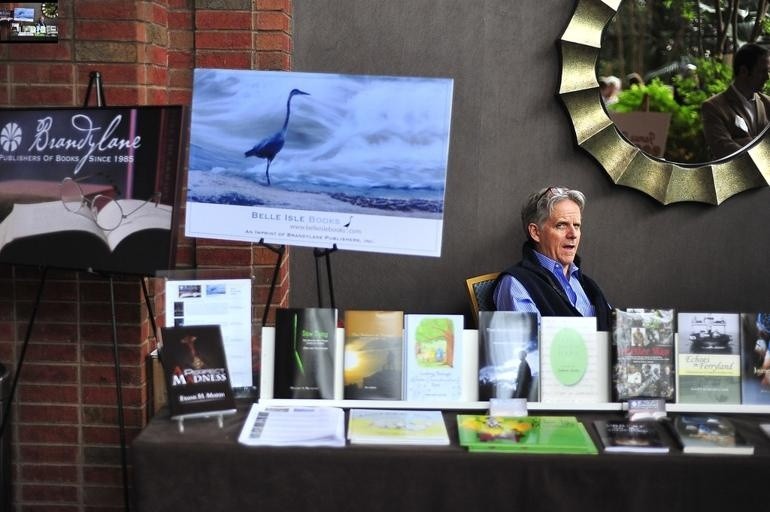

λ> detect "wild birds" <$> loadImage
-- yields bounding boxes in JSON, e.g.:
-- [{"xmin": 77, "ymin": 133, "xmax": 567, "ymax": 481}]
[{"xmin": 243, "ymin": 87, "xmax": 311, "ymax": 185}]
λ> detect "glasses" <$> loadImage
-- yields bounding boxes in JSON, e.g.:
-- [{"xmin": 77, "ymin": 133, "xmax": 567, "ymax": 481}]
[
  {"xmin": 60, "ymin": 172, "xmax": 162, "ymax": 231},
  {"xmin": 536, "ymin": 187, "xmax": 564, "ymax": 206}
]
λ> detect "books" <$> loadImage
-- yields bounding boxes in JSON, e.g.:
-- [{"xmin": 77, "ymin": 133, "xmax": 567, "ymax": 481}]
[
  {"xmin": 0, "ymin": 199, "xmax": 172, "ymax": 259},
  {"xmin": 0, "ymin": 16, "xmax": 58, "ymax": 37},
  {"xmin": 159, "ymin": 324, "xmax": 239, "ymax": 421}
]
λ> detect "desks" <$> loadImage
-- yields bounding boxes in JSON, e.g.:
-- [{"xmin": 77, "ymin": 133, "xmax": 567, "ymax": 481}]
[{"xmin": 129, "ymin": 390, "xmax": 770, "ymax": 512}]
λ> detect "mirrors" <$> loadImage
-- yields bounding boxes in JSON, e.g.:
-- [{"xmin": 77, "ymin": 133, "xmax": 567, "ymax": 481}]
[{"xmin": 559, "ymin": 0, "xmax": 770, "ymax": 207}]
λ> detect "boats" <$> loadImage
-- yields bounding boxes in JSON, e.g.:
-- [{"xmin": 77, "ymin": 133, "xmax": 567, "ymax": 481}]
[{"xmin": 688, "ymin": 315, "xmax": 733, "ymax": 353}]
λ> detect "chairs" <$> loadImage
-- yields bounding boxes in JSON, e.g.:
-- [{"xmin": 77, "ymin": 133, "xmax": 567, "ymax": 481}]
[{"xmin": 465, "ymin": 270, "xmax": 503, "ymax": 325}]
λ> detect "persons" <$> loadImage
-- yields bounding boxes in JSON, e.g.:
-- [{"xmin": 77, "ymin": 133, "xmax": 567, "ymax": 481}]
[
  {"xmin": 598, "ymin": 61, "xmax": 697, "ymax": 106},
  {"xmin": 489, "ymin": 183, "xmax": 613, "ymax": 330},
  {"xmin": 700, "ymin": 43, "xmax": 770, "ymax": 162},
  {"xmin": 701, "ymin": 33, "xmax": 733, "ymax": 54}
]
